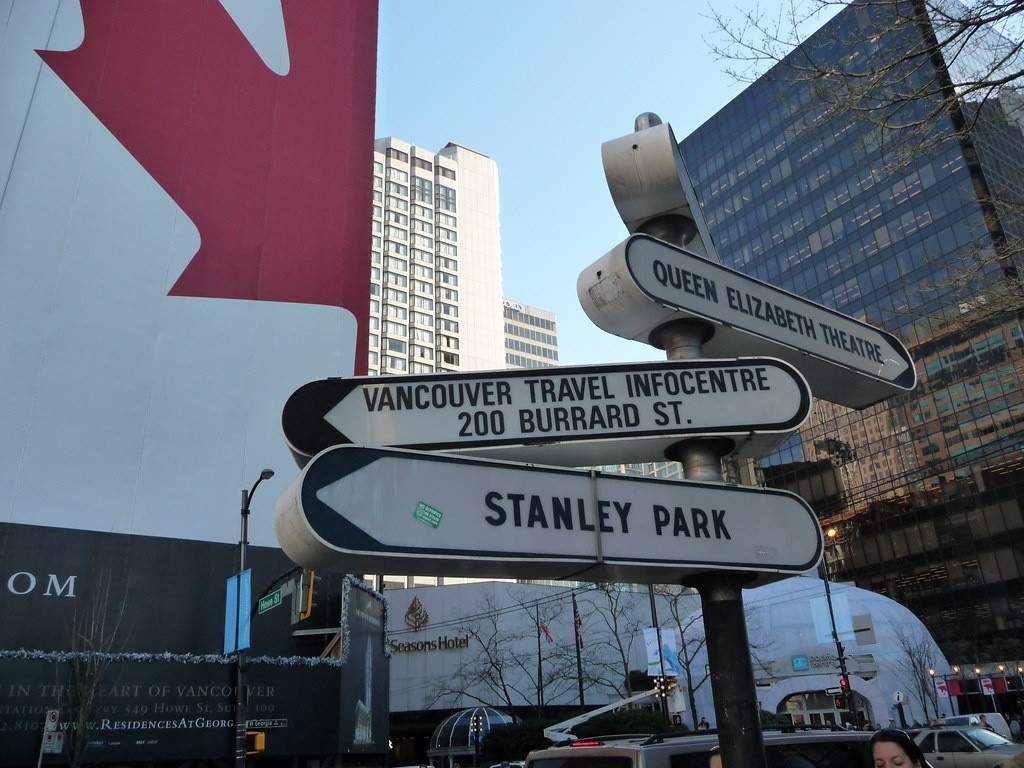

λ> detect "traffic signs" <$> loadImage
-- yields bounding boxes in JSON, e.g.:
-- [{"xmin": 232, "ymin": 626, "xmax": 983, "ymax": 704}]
[
  {"xmin": 279, "ymin": 352, "xmax": 813, "ymax": 471},
  {"xmin": 272, "ymin": 441, "xmax": 824, "ymax": 589},
  {"xmin": 575, "ymin": 232, "xmax": 919, "ymax": 412}
]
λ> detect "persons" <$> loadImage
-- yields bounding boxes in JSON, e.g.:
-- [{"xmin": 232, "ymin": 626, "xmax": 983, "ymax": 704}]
[
  {"xmin": 826, "ymin": 714, "xmax": 1024, "ymax": 743},
  {"xmin": 868, "ymin": 728, "xmax": 930, "ymax": 768},
  {"xmin": 698, "ymin": 717, "xmax": 709, "ymax": 729},
  {"xmin": 710, "ymin": 746, "xmax": 723, "ymax": 768}
]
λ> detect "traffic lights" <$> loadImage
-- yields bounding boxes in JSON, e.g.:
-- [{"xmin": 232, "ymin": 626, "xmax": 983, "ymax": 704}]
[
  {"xmin": 840, "ymin": 679, "xmax": 850, "ymax": 698},
  {"xmin": 299, "ymin": 570, "xmax": 322, "ymax": 621},
  {"xmin": 246, "ymin": 731, "xmax": 265, "ymax": 755}
]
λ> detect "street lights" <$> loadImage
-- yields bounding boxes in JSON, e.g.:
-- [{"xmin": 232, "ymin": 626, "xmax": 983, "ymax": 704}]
[
  {"xmin": 929, "ymin": 666, "xmax": 960, "ymax": 717},
  {"xmin": 234, "ymin": 468, "xmax": 276, "ymax": 768},
  {"xmin": 975, "ymin": 665, "xmax": 1006, "ymax": 713},
  {"xmin": 821, "ymin": 528, "xmax": 862, "ymax": 732}
]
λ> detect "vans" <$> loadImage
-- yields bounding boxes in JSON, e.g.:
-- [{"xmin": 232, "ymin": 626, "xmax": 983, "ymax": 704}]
[{"xmin": 945, "ymin": 711, "xmax": 1013, "ymax": 741}]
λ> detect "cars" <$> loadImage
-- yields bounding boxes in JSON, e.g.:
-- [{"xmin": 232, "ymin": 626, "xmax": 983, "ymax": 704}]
[{"xmin": 913, "ymin": 723, "xmax": 1024, "ymax": 768}]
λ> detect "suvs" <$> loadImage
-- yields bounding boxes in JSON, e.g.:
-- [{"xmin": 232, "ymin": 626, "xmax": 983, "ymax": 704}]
[{"xmin": 523, "ymin": 718, "xmax": 936, "ymax": 768}]
